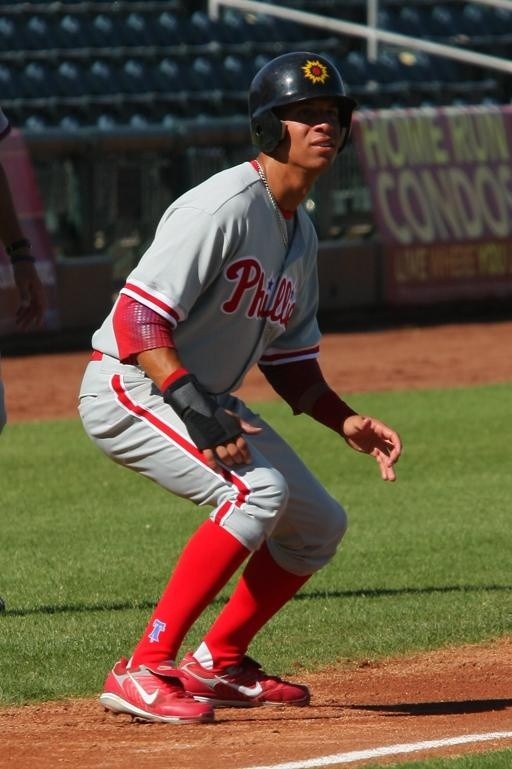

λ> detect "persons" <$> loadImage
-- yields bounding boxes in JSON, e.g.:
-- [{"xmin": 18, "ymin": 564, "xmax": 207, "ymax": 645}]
[
  {"xmin": 72, "ymin": 48, "xmax": 404, "ymax": 726},
  {"xmin": 0, "ymin": 115, "xmax": 61, "ymax": 287},
  {"xmin": 0, "ymin": 165, "xmax": 46, "ymax": 336}
]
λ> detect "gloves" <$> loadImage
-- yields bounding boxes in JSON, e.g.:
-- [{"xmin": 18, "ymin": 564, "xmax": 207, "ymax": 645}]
[{"xmin": 163, "ymin": 373, "xmax": 245, "ymax": 452}]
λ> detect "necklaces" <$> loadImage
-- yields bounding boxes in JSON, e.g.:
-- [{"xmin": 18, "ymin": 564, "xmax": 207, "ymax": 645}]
[{"xmin": 255, "ymin": 160, "xmax": 290, "ymax": 248}]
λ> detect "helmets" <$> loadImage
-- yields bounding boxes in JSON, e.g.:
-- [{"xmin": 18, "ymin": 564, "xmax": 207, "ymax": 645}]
[{"xmin": 247, "ymin": 51, "xmax": 358, "ymax": 155}]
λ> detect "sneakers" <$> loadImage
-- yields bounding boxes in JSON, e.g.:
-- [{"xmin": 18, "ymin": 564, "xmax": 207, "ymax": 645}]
[
  {"xmin": 99, "ymin": 656, "xmax": 215, "ymax": 725},
  {"xmin": 177, "ymin": 652, "xmax": 310, "ymax": 708}
]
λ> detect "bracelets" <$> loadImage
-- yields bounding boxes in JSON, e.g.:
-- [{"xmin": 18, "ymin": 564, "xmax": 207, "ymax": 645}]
[
  {"xmin": 11, "ymin": 255, "xmax": 35, "ymax": 265},
  {"xmin": 4, "ymin": 238, "xmax": 32, "ymax": 256}
]
